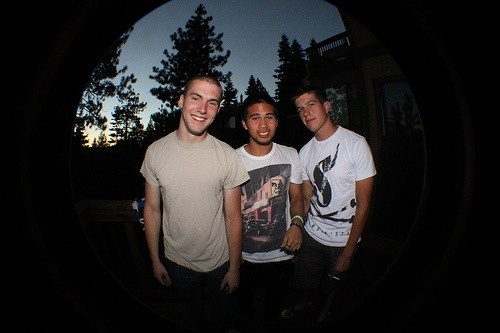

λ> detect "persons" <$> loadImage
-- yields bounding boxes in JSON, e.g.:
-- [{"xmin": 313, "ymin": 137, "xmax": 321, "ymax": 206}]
[
  {"xmin": 233, "ymin": 93, "xmax": 304, "ymax": 333},
  {"xmin": 139, "ymin": 75, "xmax": 251, "ymax": 333},
  {"xmin": 280, "ymin": 86, "xmax": 377, "ymax": 320}
]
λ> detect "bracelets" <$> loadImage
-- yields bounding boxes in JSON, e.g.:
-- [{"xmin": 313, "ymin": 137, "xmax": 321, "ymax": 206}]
[{"xmin": 290, "ymin": 215, "xmax": 304, "ymax": 232}]
